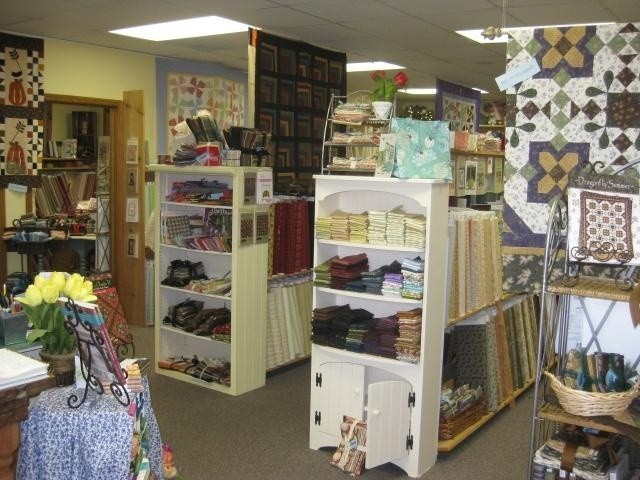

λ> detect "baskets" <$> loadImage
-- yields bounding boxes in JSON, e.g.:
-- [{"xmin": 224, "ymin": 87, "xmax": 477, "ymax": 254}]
[
  {"xmin": 543, "ymin": 369, "xmax": 640, "ymax": 417},
  {"xmin": 438, "ymin": 397, "xmax": 485, "ymax": 440}
]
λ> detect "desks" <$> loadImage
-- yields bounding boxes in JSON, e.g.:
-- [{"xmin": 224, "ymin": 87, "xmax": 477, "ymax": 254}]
[{"xmin": 19, "ymin": 347, "xmax": 149, "ymax": 480}]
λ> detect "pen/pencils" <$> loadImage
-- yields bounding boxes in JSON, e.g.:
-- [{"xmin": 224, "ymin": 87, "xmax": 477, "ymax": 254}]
[{"xmin": 0, "ymin": 283, "xmax": 20, "ymax": 313}]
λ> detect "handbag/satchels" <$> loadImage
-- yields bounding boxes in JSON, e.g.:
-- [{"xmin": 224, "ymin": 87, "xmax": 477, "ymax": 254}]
[{"xmin": 387, "ymin": 116, "xmax": 454, "ymax": 182}]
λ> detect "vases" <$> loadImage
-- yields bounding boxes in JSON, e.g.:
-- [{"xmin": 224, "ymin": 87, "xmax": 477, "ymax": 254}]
[{"xmin": 40, "ymin": 349, "xmax": 78, "ymax": 388}]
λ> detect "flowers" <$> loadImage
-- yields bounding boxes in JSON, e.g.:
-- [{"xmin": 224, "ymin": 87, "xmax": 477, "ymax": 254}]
[
  {"xmin": 366, "ymin": 70, "xmax": 409, "ymax": 102},
  {"xmin": 14, "ymin": 270, "xmax": 97, "ymax": 356}
]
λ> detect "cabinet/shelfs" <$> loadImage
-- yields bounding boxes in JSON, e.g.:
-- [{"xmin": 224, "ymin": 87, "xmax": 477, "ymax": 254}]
[
  {"xmin": 528, "ymin": 157, "xmax": 640, "ymax": 480},
  {"xmin": 307, "ymin": 90, "xmax": 556, "ymax": 479},
  {"xmin": 147, "ymin": 162, "xmax": 315, "ymax": 396}
]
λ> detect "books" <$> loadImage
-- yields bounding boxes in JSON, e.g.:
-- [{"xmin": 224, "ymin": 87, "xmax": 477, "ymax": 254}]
[
  {"xmin": 57, "ymin": 296, "xmax": 150, "ymax": 394},
  {"xmin": 159, "ymin": 208, "xmax": 232, "ymax": 252},
  {"xmin": 35, "ymin": 139, "xmax": 96, "ymax": 218}
]
